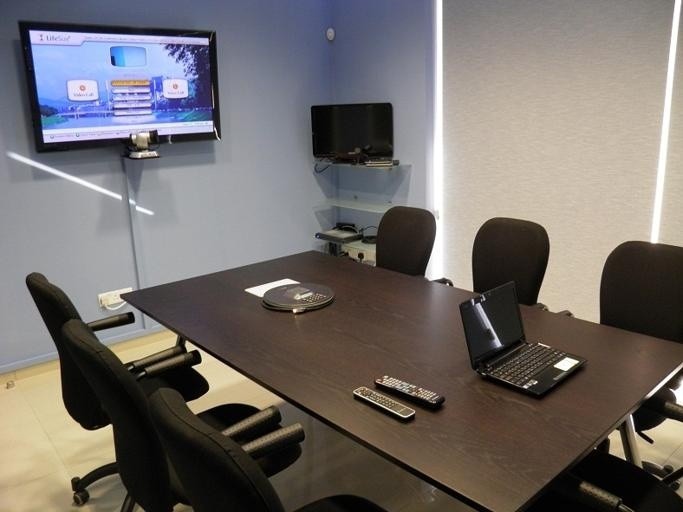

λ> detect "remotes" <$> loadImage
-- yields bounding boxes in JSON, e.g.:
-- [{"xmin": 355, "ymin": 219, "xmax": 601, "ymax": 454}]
[
  {"xmin": 353, "ymin": 386, "xmax": 415, "ymax": 419},
  {"xmin": 374, "ymin": 374, "xmax": 446, "ymax": 405}
]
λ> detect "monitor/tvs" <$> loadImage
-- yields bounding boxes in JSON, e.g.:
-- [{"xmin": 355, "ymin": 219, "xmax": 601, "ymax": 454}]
[
  {"xmin": 313, "ymin": 103, "xmax": 393, "ymax": 163},
  {"xmin": 17, "ymin": 20, "xmax": 221, "ymax": 152}
]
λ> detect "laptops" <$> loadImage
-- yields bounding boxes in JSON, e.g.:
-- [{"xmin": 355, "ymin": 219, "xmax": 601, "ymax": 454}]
[{"xmin": 460, "ymin": 281, "xmax": 588, "ymax": 396}]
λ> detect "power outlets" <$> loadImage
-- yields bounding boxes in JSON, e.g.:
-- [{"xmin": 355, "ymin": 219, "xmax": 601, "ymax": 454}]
[{"xmin": 98, "ymin": 287, "xmax": 132, "ymax": 309}]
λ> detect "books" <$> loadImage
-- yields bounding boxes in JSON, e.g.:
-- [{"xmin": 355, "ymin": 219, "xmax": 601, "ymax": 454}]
[{"xmin": 362, "ymin": 157, "xmax": 393, "ymax": 166}]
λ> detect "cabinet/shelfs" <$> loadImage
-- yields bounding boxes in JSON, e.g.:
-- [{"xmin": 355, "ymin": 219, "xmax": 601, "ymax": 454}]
[{"xmin": 312, "ymin": 160, "xmax": 411, "ymax": 264}]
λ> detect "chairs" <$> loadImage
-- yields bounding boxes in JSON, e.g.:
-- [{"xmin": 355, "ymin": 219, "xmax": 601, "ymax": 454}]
[
  {"xmin": 428, "ymin": 216, "xmax": 549, "ymax": 310},
  {"xmin": 25, "ymin": 272, "xmax": 209, "ymax": 506},
  {"xmin": 555, "ymin": 241, "xmax": 683, "ymax": 444},
  {"xmin": 149, "ymin": 389, "xmax": 391, "ymax": 511},
  {"xmin": 62, "ymin": 319, "xmax": 302, "ymax": 512},
  {"xmin": 376, "ymin": 206, "xmax": 436, "ymax": 274}
]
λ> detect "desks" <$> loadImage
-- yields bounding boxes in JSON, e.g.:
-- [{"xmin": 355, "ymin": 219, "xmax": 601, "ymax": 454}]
[{"xmin": 121, "ymin": 251, "xmax": 683, "ymax": 512}]
